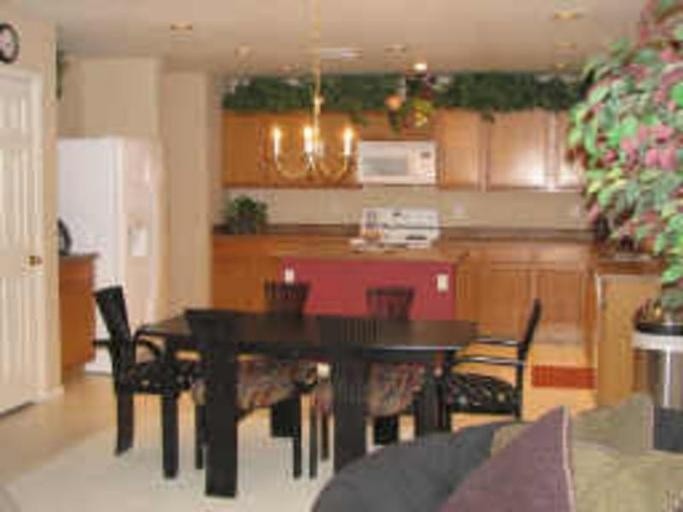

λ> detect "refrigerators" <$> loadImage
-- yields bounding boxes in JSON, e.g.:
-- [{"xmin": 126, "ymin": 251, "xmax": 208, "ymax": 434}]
[{"xmin": 59, "ymin": 134, "xmax": 160, "ymax": 341}]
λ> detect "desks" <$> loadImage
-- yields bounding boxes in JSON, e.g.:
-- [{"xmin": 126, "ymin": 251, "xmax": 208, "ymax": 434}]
[{"xmin": 139, "ymin": 308, "xmax": 474, "ymax": 499}]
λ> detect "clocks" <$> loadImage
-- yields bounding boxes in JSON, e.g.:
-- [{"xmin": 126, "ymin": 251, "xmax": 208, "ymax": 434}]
[{"xmin": 0, "ymin": 22, "xmax": 21, "ymax": 64}]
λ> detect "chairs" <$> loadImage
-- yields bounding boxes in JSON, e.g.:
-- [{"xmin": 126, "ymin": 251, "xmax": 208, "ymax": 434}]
[
  {"xmin": 182, "ymin": 280, "xmax": 414, "ymax": 500},
  {"xmin": 93, "ymin": 284, "xmax": 206, "ymax": 472},
  {"xmin": 443, "ymin": 298, "xmax": 542, "ymax": 429}
]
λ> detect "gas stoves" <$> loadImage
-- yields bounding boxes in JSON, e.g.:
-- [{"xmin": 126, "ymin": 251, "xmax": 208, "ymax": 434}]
[{"xmin": 350, "ymin": 232, "xmax": 439, "ymax": 253}]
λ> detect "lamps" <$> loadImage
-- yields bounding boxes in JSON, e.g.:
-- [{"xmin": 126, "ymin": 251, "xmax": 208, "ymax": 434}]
[{"xmin": 269, "ymin": 0, "xmax": 355, "ymax": 182}]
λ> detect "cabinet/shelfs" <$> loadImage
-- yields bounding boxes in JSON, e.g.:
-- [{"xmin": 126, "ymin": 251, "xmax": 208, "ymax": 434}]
[
  {"xmin": 210, "ymin": 234, "xmax": 269, "ymax": 314},
  {"xmin": 435, "ymin": 106, "xmax": 486, "ymax": 192},
  {"xmin": 477, "ymin": 240, "xmax": 585, "ymax": 345},
  {"xmin": 359, "ymin": 106, "xmax": 434, "ymax": 140},
  {"xmin": 220, "ymin": 109, "xmax": 264, "ymax": 187},
  {"xmin": 584, "ymin": 260, "xmax": 664, "ymax": 407},
  {"xmin": 487, "ymin": 105, "xmax": 588, "ymax": 193},
  {"xmin": 265, "ymin": 108, "xmax": 364, "ymax": 189}
]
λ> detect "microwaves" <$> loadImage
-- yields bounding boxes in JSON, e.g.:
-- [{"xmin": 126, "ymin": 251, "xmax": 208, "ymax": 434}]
[{"xmin": 357, "ymin": 142, "xmax": 434, "ymax": 184}]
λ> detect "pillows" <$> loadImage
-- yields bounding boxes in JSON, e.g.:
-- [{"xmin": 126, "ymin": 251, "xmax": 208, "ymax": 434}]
[{"xmin": 439, "ymin": 391, "xmax": 683, "ymax": 512}]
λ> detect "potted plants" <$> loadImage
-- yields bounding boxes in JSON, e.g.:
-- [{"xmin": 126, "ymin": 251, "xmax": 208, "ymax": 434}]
[
  {"xmin": 218, "ymin": 193, "xmax": 269, "ymax": 235},
  {"xmin": 565, "ymin": 2, "xmax": 682, "ymax": 410}
]
